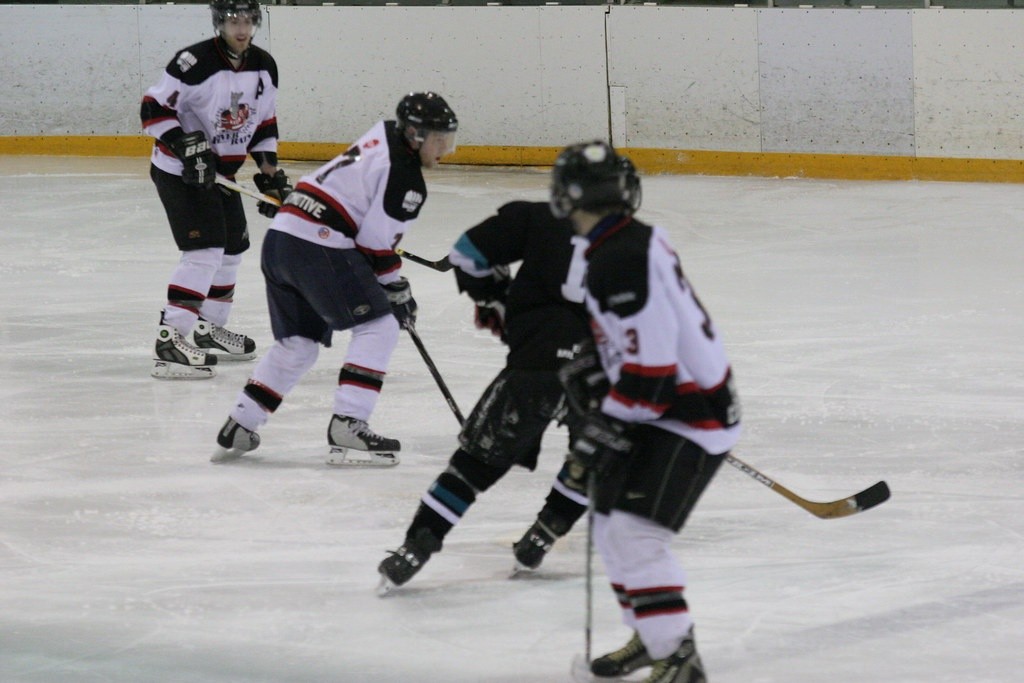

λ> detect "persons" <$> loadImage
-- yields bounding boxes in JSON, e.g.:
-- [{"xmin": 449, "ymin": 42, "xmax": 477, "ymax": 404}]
[
  {"xmin": 548, "ymin": 138, "xmax": 746, "ymax": 683},
  {"xmin": 378, "ymin": 144, "xmax": 644, "ymax": 587},
  {"xmin": 143, "ymin": 0, "xmax": 293, "ymax": 369},
  {"xmin": 217, "ymin": 88, "xmax": 459, "ymax": 452}
]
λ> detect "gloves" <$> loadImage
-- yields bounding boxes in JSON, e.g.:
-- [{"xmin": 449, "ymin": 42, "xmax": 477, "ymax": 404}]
[
  {"xmin": 469, "ymin": 282, "xmax": 509, "ymax": 344},
  {"xmin": 379, "ymin": 276, "xmax": 417, "ymax": 329},
  {"xmin": 170, "ymin": 130, "xmax": 214, "ymax": 192},
  {"xmin": 568, "ymin": 407, "xmax": 634, "ymax": 474},
  {"xmin": 552, "ymin": 340, "xmax": 611, "ymax": 417},
  {"xmin": 254, "ymin": 168, "xmax": 293, "ymax": 219}
]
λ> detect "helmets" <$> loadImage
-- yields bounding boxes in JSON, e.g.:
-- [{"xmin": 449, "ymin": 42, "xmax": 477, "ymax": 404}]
[
  {"xmin": 209, "ymin": 0, "xmax": 263, "ymax": 37},
  {"xmin": 618, "ymin": 157, "xmax": 641, "ymax": 219},
  {"xmin": 395, "ymin": 91, "xmax": 459, "ymax": 157},
  {"xmin": 550, "ymin": 142, "xmax": 621, "ymax": 219}
]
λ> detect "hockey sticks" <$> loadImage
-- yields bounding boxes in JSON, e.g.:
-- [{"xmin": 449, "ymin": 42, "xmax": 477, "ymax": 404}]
[
  {"xmin": 213, "ymin": 172, "xmax": 452, "ymax": 273},
  {"xmin": 405, "ymin": 321, "xmax": 465, "ymax": 425},
  {"xmin": 584, "ymin": 472, "xmax": 598, "ymax": 664},
  {"xmin": 722, "ymin": 450, "xmax": 892, "ymax": 521}
]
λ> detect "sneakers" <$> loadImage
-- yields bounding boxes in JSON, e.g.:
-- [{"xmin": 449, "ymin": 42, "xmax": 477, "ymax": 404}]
[
  {"xmin": 643, "ymin": 624, "xmax": 705, "ymax": 683},
  {"xmin": 192, "ymin": 314, "xmax": 256, "ymax": 361},
  {"xmin": 326, "ymin": 414, "xmax": 401, "ymax": 467},
  {"xmin": 508, "ymin": 508, "xmax": 572, "ymax": 578},
  {"xmin": 595, "ymin": 628, "xmax": 657, "ymax": 683},
  {"xmin": 208, "ymin": 406, "xmax": 260, "ymax": 464},
  {"xmin": 151, "ymin": 309, "xmax": 218, "ymax": 380},
  {"xmin": 375, "ymin": 526, "xmax": 442, "ymax": 597}
]
